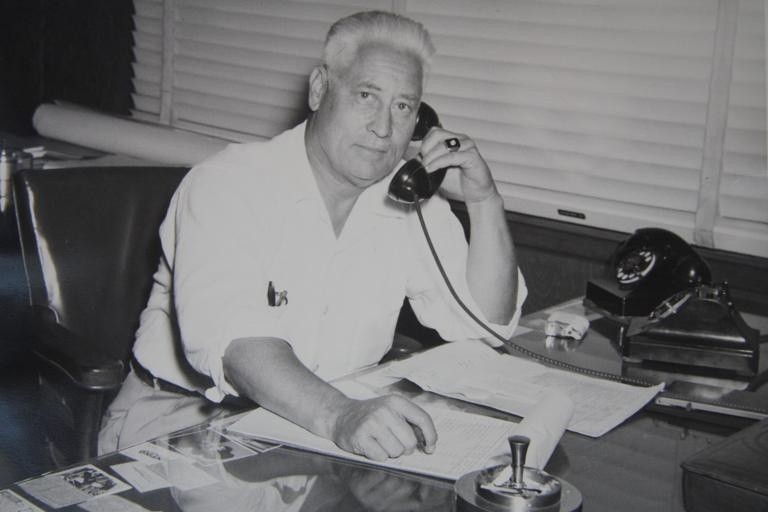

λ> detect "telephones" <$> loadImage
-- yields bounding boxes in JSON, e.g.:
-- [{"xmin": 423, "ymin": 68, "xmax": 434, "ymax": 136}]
[
  {"xmin": 386, "ymin": 101, "xmax": 449, "ymax": 205},
  {"xmin": 584, "ymin": 227, "xmax": 712, "ymax": 317}
]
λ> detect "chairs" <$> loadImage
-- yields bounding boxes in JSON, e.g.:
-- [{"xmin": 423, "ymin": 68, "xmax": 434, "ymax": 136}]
[{"xmin": 10, "ymin": 164, "xmax": 200, "ymax": 471}]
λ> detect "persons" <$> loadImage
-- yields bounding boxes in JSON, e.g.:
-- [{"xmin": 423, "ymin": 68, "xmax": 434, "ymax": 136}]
[
  {"xmin": 85, "ymin": 9, "xmax": 519, "ymax": 464},
  {"xmin": 157, "ymin": 434, "xmax": 432, "ymax": 512}
]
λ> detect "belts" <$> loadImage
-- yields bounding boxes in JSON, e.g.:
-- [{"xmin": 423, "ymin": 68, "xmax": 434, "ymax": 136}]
[{"xmin": 127, "ymin": 354, "xmax": 204, "ymax": 401}]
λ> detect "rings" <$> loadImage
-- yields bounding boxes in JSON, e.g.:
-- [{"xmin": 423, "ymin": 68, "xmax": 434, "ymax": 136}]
[{"xmin": 444, "ymin": 139, "xmax": 460, "ymax": 153}]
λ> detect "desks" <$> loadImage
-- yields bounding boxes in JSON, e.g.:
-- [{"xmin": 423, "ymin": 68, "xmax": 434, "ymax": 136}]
[{"xmin": 4, "ymin": 294, "xmax": 765, "ymax": 509}]
